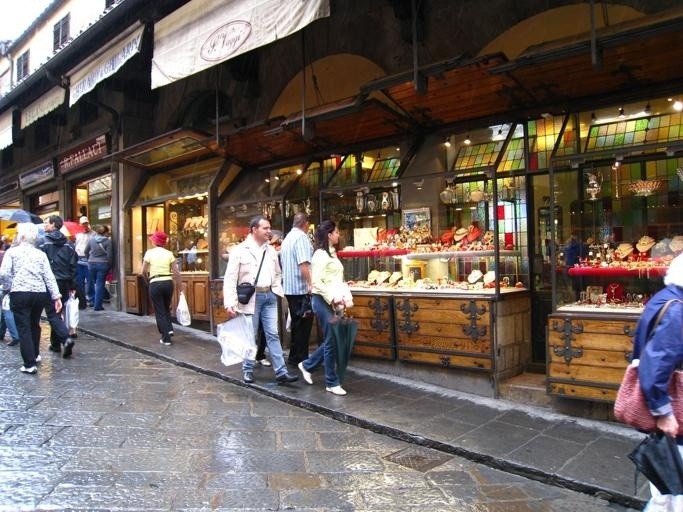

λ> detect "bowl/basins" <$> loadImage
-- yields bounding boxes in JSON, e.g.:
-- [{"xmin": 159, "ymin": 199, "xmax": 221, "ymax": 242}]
[{"xmin": 627, "ymin": 180, "xmax": 668, "ymax": 194}]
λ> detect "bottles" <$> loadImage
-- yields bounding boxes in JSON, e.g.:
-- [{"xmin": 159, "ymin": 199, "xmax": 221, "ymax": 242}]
[{"xmin": 501, "ymin": 184, "xmax": 507, "ymax": 199}]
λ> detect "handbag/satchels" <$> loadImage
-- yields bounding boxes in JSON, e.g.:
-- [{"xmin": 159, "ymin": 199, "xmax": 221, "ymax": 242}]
[
  {"xmin": 216, "ymin": 315, "xmax": 257, "ymax": 367},
  {"xmin": 65, "ymin": 293, "xmax": 80, "ymax": 329},
  {"xmin": 614, "ymin": 360, "xmax": 683, "ymax": 439},
  {"xmin": 237, "ymin": 282, "xmax": 255, "ymax": 304}
]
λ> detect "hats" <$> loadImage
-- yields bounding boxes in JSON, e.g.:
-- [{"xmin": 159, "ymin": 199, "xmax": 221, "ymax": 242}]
[
  {"xmin": 79, "ymin": 216, "xmax": 89, "ymax": 224},
  {"xmin": 149, "ymin": 230, "xmax": 168, "ymax": 247}
]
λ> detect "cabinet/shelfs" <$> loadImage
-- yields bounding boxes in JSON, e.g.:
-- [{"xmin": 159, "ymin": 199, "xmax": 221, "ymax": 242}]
[
  {"xmin": 217, "ymin": 206, "xmax": 281, "ymax": 274},
  {"xmin": 442, "ymin": 176, "xmax": 494, "ymax": 208},
  {"xmin": 329, "ymin": 190, "xmax": 403, "ymax": 290},
  {"xmin": 556, "ymin": 164, "xmax": 682, "ymax": 314},
  {"xmin": 174, "ymin": 202, "xmax": 213, "ymax": 274},
  {"xmin": 405, "ymin": 226, "xmax": 521, "ymax": 290}
]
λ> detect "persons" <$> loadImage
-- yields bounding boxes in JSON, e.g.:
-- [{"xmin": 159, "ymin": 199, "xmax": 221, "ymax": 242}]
[
  {"xmin": 255, "ymin": 230, "xmax": 287, "ymax": 367},
  {"xmin": 630, "ymin": 249, "xmax": 683, "ymax": 512},
  {"xmin": 221, "ymin": 216, "xmax": 299, "ymax": 385},
  {"xmin": 277, "ymin": 211, "xmax": 320, "ymax": 371},
  {"xmin": 295, "ymin": 218, "xmax": 354, "ymax": 396},
  {"xmin": 0, "ymin": 206, "xmax": 116, "ymax": 373},
  {"xmin": 140, "ymin": 231, "xmax": 185, "ymax": 347}
]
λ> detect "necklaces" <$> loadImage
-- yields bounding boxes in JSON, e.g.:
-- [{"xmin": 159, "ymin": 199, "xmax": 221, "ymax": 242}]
[
  {"xmin": 362, "ymin": 225, "xmax": 495, "ymax": 290},
  {"xmin": 572, "ymin": 236, "xmax": 683, "ymax": 310}
]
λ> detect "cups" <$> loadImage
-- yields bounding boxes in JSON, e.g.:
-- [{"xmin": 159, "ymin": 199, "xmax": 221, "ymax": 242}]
[{"xmin": 470, "ymin": 192, "xmax": 483, "ymax": 202}]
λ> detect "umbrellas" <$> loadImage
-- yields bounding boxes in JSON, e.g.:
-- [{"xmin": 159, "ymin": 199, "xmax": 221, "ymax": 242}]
[
  {"xmin": 627, "ymin": 428, "xmax": 682, "ymax": 495},
  {"xmin": 328, "ymin": 300, "xmax": 358, "ymax": 387}
]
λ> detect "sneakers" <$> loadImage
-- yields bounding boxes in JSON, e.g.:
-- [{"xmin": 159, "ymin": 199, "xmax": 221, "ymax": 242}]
[
  {"xmin": 168, "ymin": 331, "xmax": 174, "ymax": 336},
  {"xmin": 7, "ymin": 340, "xmax": 19, "ymax": 346},
  {"xmin": 103, "ymin": 298, "xmax": 111, "ymax": 302},
  {"xmin": 36, "ymin": 356, "xmax": 41, "ymax": 362},
  {"xmin": 94, "ymin": 307, "xmax": 103, "ymax": 310},
  {"xmin": 49, "ymin": 345, "xmax": 61, "ymax": 352},
  {"xmin": 87, "ymin": 301, "xmax": 95, "ymax": 306},
  {"xmin": 20, "ymin": 366, "xmax": 37, "ymax": 374},
  {"xmin": 160, "ymin": 339, "xmax": 172, "ymax": 346},
  {"xmin": 69, "ymin": 334, "xmax": 77, "ymax": 338},
  {"xmin": 63, "ymin": 338, "xmax": 74, "ymax": 358},
  {"xmin": 244, "ymin": 359, "xmax": 348, "ymax": 396}
]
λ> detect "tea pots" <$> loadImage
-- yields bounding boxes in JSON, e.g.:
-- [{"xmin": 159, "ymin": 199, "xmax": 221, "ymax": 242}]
[{"xmin": 586, "ymin": 172, "xmax": 603, "ymax": 200}]
